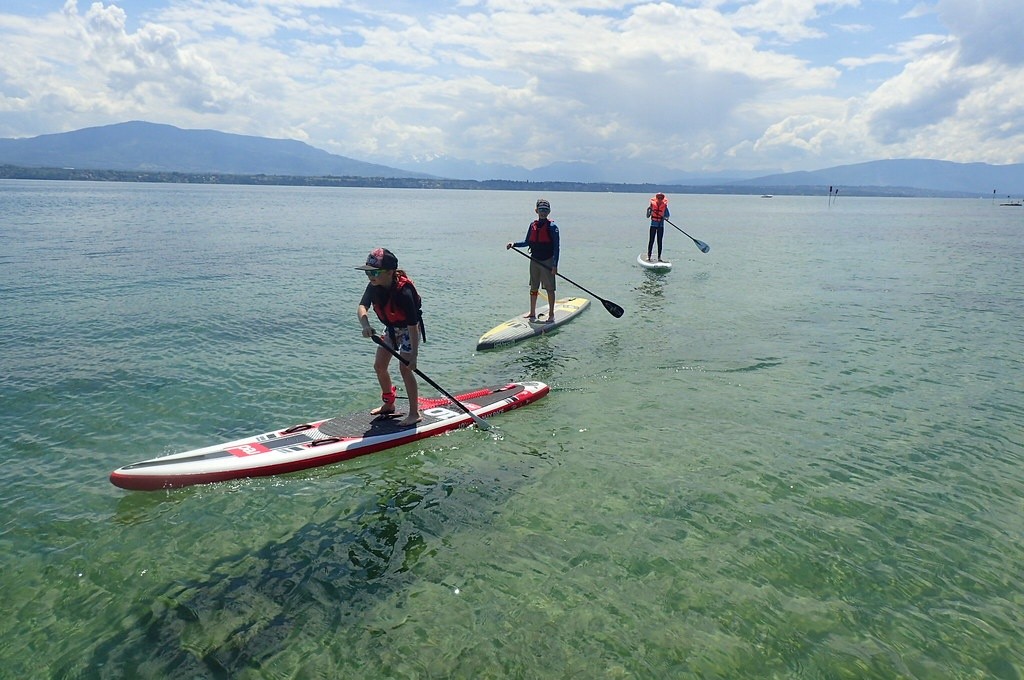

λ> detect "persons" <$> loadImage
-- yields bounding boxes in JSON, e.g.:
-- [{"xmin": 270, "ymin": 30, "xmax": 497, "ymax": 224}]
[
  {"xmin": 646, "ymin": 191, "xmax": 670, "ymax": 262},
  {"xmin": 505, "ymin": 200, "xmax": 561, "ymax": 322},
  {"xmin": 353, "ymin": 248, "xmax": 423, "ymax": 426}
]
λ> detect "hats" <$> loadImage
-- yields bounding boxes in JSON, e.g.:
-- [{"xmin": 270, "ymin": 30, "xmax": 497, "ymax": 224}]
[
  {"xmin": 536, "ymin": 199, "xmax": 550, "ymax": 210},
  {"xmin": 354, "ymin": 247, "xmax": 397, "ymax": 270},
  {"xmin": 656, "ymin": 193, "xmax": 664, "ymax": 199}
]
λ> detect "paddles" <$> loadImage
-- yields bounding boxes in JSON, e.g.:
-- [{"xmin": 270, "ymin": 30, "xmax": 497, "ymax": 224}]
[
  {"xmin": 654, "ymin": 211, "xmax": 710, "ymax": 253},
  {"xmin": 371, "ymin": 330, "xmax": 494, "ymax": 430},
  {"xmin": 510, "ymin": 243, "xmax": 624, "ymax": 318}
]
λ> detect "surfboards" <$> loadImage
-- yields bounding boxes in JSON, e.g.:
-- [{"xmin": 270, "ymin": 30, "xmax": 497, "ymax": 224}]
[
  {"xmin": 638, "ymin": 252, "xmax": 672, "ymax": 270},
  {"xmin": 109, "ymin": 380, "xmax": 550, "ymax": 489},
  {"xmin": 476, "ymin": 295, "xmax": 591, "ymax": 351}
]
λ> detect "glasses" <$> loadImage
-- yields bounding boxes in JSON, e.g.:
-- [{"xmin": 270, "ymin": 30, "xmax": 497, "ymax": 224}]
[
  {"xmin": 365, "ymin": 269, "xmax": 388, "ymax": 276},
  {"xmin": 537, "ymin": 208, "xmax": 549, "ymax": 212}
]
